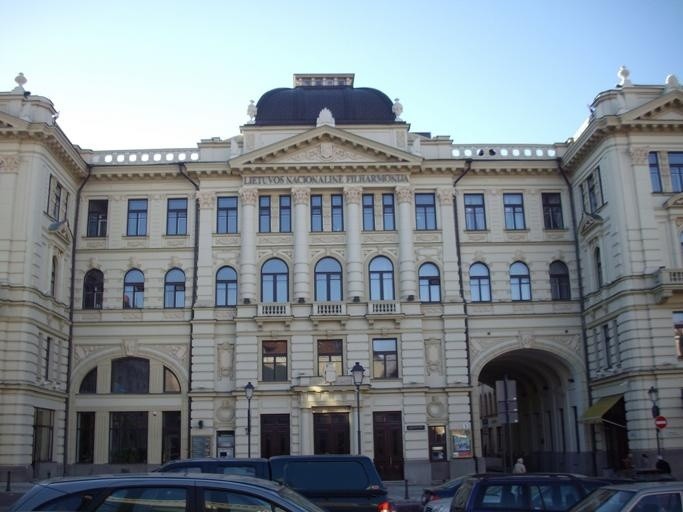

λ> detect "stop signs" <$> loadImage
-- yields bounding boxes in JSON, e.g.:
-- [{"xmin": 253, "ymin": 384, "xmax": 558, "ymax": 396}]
[{"xmin": 654, "ymin": 416, "xmax": 668, "ymax": 429}]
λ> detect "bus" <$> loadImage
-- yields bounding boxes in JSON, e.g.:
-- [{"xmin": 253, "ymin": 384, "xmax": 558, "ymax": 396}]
[{"xmin": 566, "ymin": 480, "xmax": 682, "ymax": 512}]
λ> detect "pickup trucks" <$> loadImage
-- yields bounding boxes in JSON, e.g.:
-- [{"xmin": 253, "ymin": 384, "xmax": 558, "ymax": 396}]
[{"xmin": 152, "ymin": 453, "xmax": 393, "ymax": 512}]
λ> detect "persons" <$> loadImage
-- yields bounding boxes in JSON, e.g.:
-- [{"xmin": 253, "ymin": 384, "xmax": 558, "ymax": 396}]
[
  {"xmin": 654, "ymin": 454, "xmax": 671, "ymax": 475},
  {"xmin": 621, "ymin": 452, "xmax": 632, "ymax": 478},
  {"xmin": 508, "ymin": 456, "xmax": 526, "ymax": 473},
  {"xmin": 632, "ymin": 454, "xmax": 650, "ymax": 481}
]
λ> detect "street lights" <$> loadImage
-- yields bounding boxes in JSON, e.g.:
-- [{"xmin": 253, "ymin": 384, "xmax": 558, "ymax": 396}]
[
  {"xmin": 347, "ymin": 359, "xmax": 367, "ymax": 455},
  {"xmin": 646, "ymin": 383, "xmax": 662, "ymax": 458},
  {"xmin": 241, "ymin": 380, "xmax": 255, "ymax": 458}
]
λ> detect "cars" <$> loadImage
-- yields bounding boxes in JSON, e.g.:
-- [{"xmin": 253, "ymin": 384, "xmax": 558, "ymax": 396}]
[
  {"xmin": 418, "ymin": 471, "xmax": 501, "ymax": 504},
  {"xmin": 422, "ymin": 471, "xmax": 594, "ymax": 512}
]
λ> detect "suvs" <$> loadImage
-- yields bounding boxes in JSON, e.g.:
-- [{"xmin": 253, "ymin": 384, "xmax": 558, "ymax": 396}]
[
  {"xmin": 446, "ymin": 471, "xmax": 610, "ymax": 512},
  {"xmin": 4, "ymin": 471, "xmax": 329, "ymax": 511}
]
